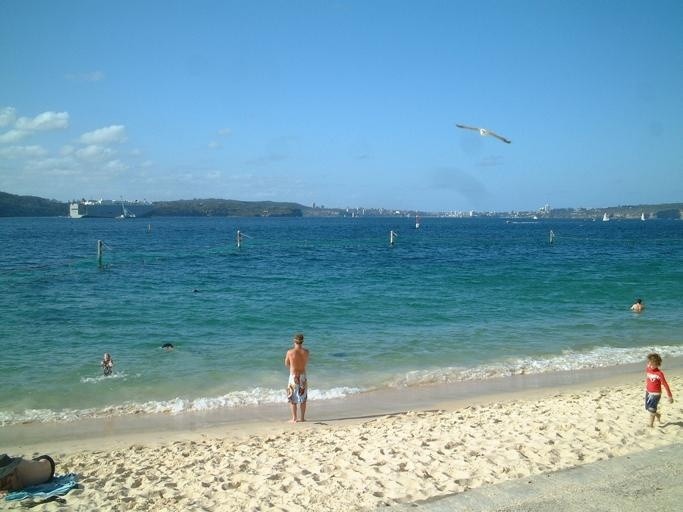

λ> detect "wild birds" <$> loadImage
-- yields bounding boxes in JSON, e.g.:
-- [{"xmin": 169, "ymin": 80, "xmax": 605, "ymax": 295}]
[{"xmin": 457, "ymin": 122, "xmax": 514, "ymax": 145}]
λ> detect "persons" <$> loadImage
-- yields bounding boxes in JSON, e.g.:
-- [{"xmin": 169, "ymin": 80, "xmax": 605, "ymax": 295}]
[
  {"xmin": 99, "ymin": 351, "xmax": 112, "ymax": 375},
  {"xmin": 284, "ymin": 334, "xmax": 309, "ymax": 422},
  {"xmin": 643, "ymin": 353, "xmax": 673, "ymax": 428},
  {"xmin": 630, "ymin": 298, "xmax": 645, "ymax": 313},
  {"xmin": 0, "ymin": 453, "xmax": 54, "ymax": 490}
]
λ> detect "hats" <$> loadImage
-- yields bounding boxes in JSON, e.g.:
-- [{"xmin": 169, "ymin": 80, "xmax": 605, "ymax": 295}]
[{"xmin": 0, "ymin": 453, "xmax": 22, "ymax": 480}]
